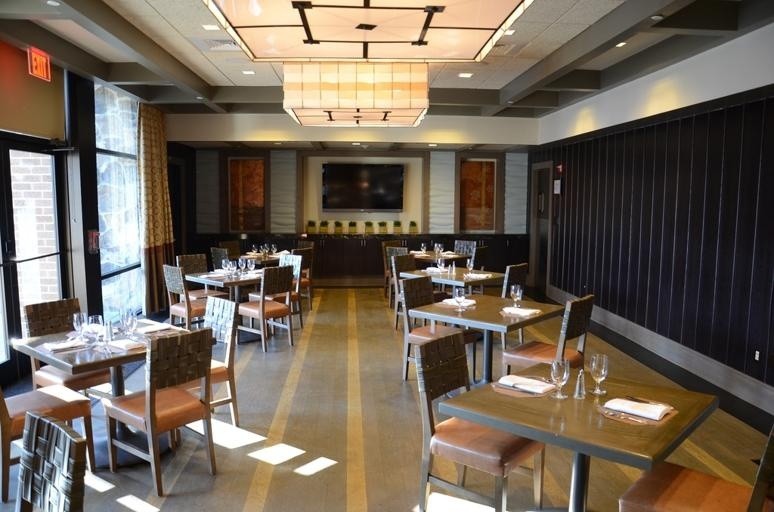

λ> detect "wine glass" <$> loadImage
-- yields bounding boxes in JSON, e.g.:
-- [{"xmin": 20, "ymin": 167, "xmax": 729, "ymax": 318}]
[
  {"xmin": 71, "ymin": 308, "xmax": 138, "ymax": 353},
  {"xmin": 420, "ymin": 242, "xmax": 443, "ymax": 254},
  {"xmin": 437, "ymin": 259, "xmax": 445, "ymax": 275},
  {"xmin": 589, "ymin": 354, "xmax": 609, "ymax": 397},
  {"xmin": 510, "ymin": 285, "xmax": 522, "ymax": 308},
  {"xmin": 250, "ymin": 244, "xmax": 278, "ymax": 257},
  {"xmin": 455, "ymin": 288, "xmax": 467, "ymax": 313},
  {"xmin": 549, "ymin": 357, "xmax": 571, "ymax": 400},
  {"xmin": 222, "ymin": 257, "xmax": 257, "ymax": 278},
  {"xmin": 466, "ymin": 258, "xmax": 474, "ymax": 274}
]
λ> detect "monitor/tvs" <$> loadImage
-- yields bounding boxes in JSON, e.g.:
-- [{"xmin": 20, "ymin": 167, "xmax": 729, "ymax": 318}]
[{"xmin": 322, "ymin": 163, "xmax": 403, "ymax": 212}]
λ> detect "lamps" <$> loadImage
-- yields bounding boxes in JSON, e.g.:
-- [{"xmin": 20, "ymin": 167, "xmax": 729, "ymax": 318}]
[{"xmin": 282, "ymin": 61, "xmax": 430, "ymax": 128}]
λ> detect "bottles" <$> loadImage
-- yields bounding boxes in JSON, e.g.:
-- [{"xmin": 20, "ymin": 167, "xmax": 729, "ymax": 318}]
[
  {"xmin": 448, "ymin": 260, "xmax": 457, "ymax": 277},
  {"xmin": 572, "ymin": 367, "xmax": 589, "ymax": 400}
]
